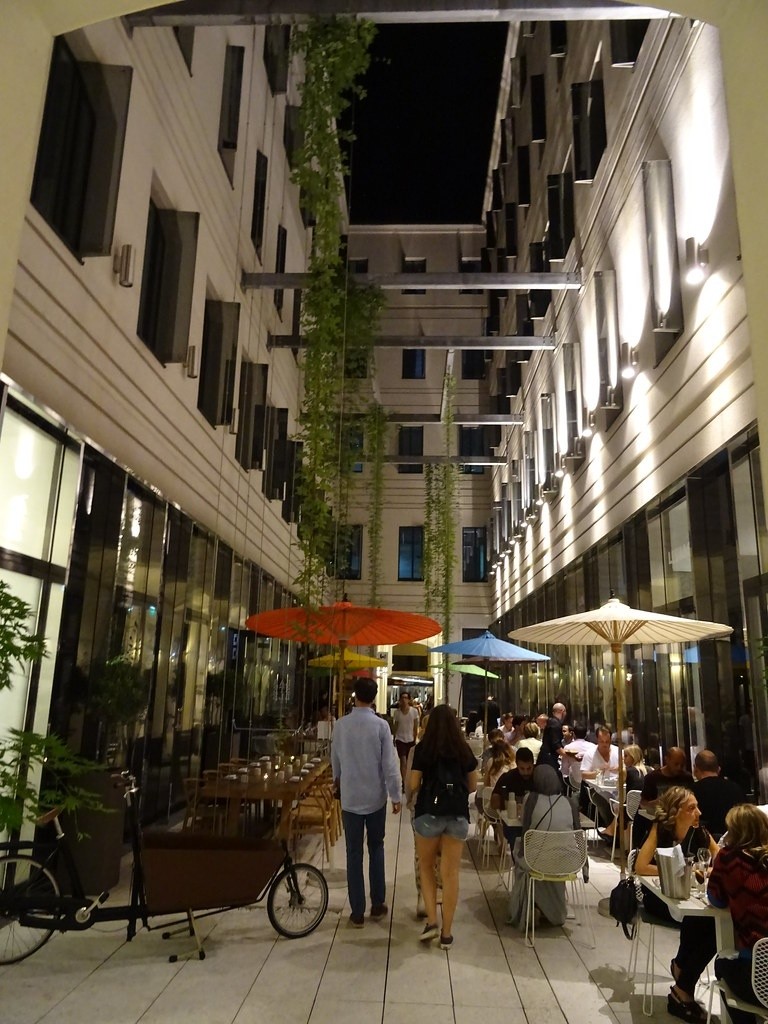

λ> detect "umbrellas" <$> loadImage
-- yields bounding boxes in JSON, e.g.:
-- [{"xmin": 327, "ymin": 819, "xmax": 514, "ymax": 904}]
[
  {"xmin": 428, "ymin": 662, "xmax": 499, "ymax": 717},
  {"xmin": 426, "ymin": 630, "xmax": 551, "ymax": 774},
  {"xmin": 452, "ymin": 656, "xmax": 544, "ymax": 717},
  {"xmin": 308, "ymin": 648, "xmax": 388, "ymax": 718},
  {"xmin": 344, "ymin": 670, "xmax": 382, "ymax": 678},
  {"xmin": 507, "ymin": 589, "xmax": 734, "ymax": 880},
  {"xmin": 245, "ymin": 592, "xmax": 442, "ymax": 718}
]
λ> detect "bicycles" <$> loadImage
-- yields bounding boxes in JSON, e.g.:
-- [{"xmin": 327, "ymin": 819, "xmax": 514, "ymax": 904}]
[{"xmin": 0, "ymin": 769, "xmax": 329, "ymax": 965}]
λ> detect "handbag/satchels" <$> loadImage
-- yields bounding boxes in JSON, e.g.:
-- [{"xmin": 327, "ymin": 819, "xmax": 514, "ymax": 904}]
[{"xmin": 609, "ymin": 849, "xmax": 639, "ymax": 940}]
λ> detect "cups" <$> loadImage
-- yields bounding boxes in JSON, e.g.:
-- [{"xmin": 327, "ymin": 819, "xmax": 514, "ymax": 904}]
[{"xmin": 241, "ymin": 753, "xmax": 307, "ymax": 782}]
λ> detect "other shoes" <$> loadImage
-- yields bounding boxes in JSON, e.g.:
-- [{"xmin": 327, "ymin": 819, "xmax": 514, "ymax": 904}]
[
  {"xmin": 349, "ymin": 913, "xmax": 365, "ymax": 928},
  {"xmin": 371, "ymin": 906, "xmax": 388, "ymax": 920},
  {"xmin": 597, "ymin": 829, "xmax": 620, "ymax": 847},
  {"xmin": 417, "ymin": 898, "xmax": 428, "ymax": 918},
  {"xmin": 436, "ymin": 888, "xmax": 442, "ymax": 903}
]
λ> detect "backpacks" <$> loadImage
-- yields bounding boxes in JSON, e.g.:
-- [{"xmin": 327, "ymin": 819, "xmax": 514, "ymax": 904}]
[{"xmin": 427, "ymin": 771, "xmax": 463, "ymax": 817}]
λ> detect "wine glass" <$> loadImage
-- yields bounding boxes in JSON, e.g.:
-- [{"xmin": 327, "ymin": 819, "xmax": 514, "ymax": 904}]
[{"xmin": 698, "ymin": 848, "xmax": 711, "ymax": 896}]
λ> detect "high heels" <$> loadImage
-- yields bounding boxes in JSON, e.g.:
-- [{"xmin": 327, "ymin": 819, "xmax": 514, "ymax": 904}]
[
  {"xmin": 671, "ymin": 958, "xmax": 677, "ymax": 982},
  {"xmin": 668, "ymin": 985, "xmax": 707, "ymax": 1024}
]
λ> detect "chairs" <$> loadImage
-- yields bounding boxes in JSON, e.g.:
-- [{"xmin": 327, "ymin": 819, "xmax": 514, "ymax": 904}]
[
  {"xmin": 468, "ymin": 740, "xmax": 768, "ymax": 1024},
  {"xmin": 182, "ymin": 754, "xmax": 340, "ymax": 862}
]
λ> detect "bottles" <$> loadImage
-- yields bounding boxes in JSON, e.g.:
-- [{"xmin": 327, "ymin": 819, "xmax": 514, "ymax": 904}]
[
  {"xmin": 597, "ymin": 772, "xmax": 602, "ymax": 784},
  {"xmin": 508, "ymin": 792, "xmax": 517, "ymax": 819}
]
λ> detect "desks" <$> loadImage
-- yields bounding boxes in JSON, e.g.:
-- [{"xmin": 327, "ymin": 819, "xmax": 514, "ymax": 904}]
[
  {"xmin": 638, "ymin": 875, "xmax": 737, "ymax": 1024},
  {"xmin": 496, "ymin": 809, "xmax": 595, "ymax": 828}
]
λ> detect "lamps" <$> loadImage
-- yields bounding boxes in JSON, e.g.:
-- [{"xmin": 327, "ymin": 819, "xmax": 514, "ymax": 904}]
[
  {"xmin": 114, "ymin": 245, "xmax": 287, "ymax": 501},
  {"xmin": 488, "ymin": 237, "xmax": 710, "ymax": 574}
]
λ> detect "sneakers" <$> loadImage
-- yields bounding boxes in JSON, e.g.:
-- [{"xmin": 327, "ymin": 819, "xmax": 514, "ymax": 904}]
[
  {"xmin": 420, "ymin": 923, "xmax": 440, "ymax": 939},
  {"xmin": 440, "ymin": 935, "xmax": 453, "ymax": 950}
]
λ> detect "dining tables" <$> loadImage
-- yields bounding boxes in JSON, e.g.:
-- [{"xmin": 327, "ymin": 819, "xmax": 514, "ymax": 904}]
[
  {"xmin": 201, "ymin": 755, "xmax": 332, "ymax": 846},
  {"xmin": 584, "ymin": 778, "xmax": 628, "ymax": 847}
]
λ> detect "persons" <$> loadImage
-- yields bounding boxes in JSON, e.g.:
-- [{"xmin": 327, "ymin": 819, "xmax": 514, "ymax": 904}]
[
  {"xmin": 393, "ymin": 692, "xmax": 418, "ymax": 794},
  {"xmin": 707, "ymin": 804, "xmax": 768, "ymax": 1024},
  {"xmin": 331, "ymin": 677, "xmax": 402, "ymax": 928},
  {"xmin": 490, "ymin": 747, "xmax": 589, "ymax": 932},
  {"xmin": 579, "ymin": 726, "xmax": 750, "ymax": 873},
  {"xmin": 635, "ymin": 786, "xmax": 720, "ymax": 1024},
  {"xmin": 467, "ymin": 691, "xmax": 597, "ymax": 857},
  {"xmin": 405, "ymin": 704, "xmax": 479, "ymax": 950}
]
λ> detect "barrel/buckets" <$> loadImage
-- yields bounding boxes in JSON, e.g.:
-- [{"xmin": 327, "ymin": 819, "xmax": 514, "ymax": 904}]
[{"xmin": 655, "ymin": 852, "xmax": 695, "ymax": 899}]
